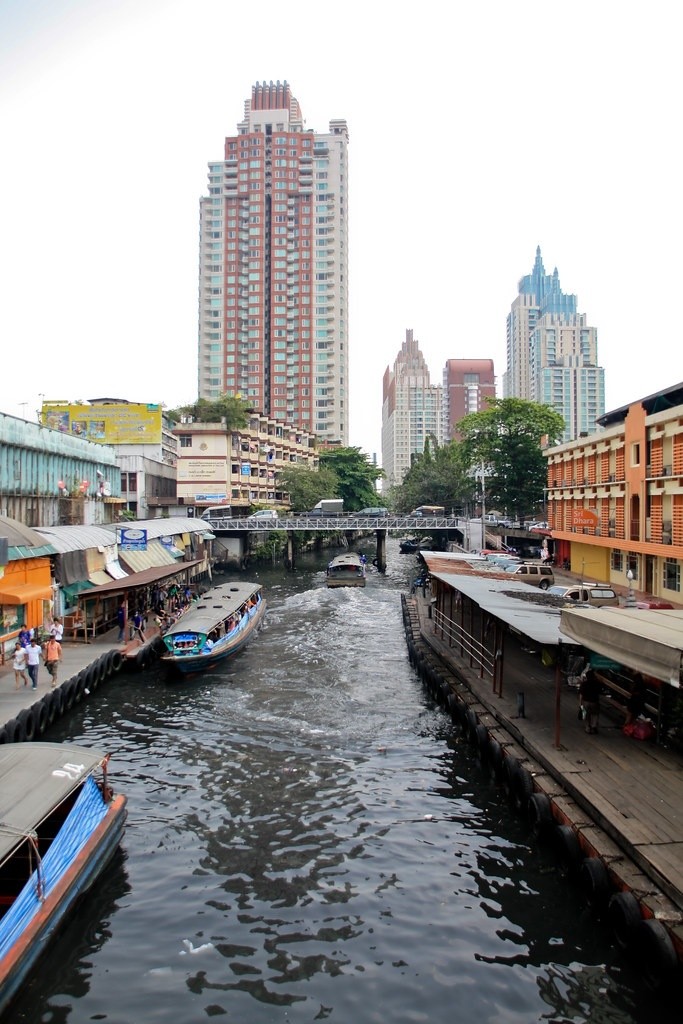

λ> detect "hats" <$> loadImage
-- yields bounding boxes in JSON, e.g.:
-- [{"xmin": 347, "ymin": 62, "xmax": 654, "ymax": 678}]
[{"xmin": 29, "ymin": 639, "xmax": 36, "ymax": 642}]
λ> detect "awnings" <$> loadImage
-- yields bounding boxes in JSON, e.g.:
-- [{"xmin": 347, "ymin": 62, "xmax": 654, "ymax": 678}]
[
  {"xmin": 0, "ymin": 584, "xmax": 48, "ymax": 604},
  {"xmin": 74, "ymin": 557, "xmax": 205, "ymax": 599}
]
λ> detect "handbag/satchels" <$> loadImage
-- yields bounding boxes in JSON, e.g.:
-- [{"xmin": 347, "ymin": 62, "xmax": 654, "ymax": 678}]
[
  {"xmin": 578, "ymin": 705, "xmax": 589, "ymax": 720},
  {"xmin": 44, "ymin": 660, "xmax": 48, "ymax": 666}
]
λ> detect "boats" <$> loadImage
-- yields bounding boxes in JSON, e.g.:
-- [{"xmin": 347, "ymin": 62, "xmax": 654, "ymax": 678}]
[
  {"xmin": 0, "ymin": 741, "xmax": 131, "ymax": 1024},
  {"xmin": 160, "ymin": 580, "xmax": 268, "ymax": 666},
  {"xmin": 326, "ymin": 551, "xmax": 367, "ymax": 588}
]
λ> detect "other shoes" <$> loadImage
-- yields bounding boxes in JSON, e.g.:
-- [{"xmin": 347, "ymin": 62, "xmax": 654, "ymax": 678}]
[
  {"xmin": 47, "ymin": 676, "xmax": 57, "ymax": 686},
  {"xmin": 32, "ymin": 687, "xmax": 37, "ymax": 691},
  {"xmin": 585, "ymin": 728, "xmax": 596, "ymax": 735}
]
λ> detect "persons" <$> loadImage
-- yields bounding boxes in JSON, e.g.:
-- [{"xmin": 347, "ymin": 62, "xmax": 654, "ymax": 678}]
[
  {"xmin": 455, "ymin": 590, "xmax": 461, "ymax": 612},
  {"xmin": 24, "ymin": 639, "xmax": 44, "ymax": 690},
  {"xmin": 579, "ymin": 671, "xmax": 599, "ymax": 734},
  {"xmin": 121, "ymin": 577, "xmax": 258, "ymax": 642},
  {"xmin": 51, "ymin": 618, "xmax": 63, "ymax": 644},
  {"xmin": 45, "ymin": 635, "xmax": 61, "ymax": 686},
  {"xmin": 329, "ymin": 555, "xmax": 366, "ymax": 576},
  {"xmin": 19, "ymin": 624, "xmax": 32, "ymax": 648},
  {"xmin": 623, "ymin": 673, "xmax": 644, "ymax": 723},
  {"xmin": 13, "ymin": 643, "xmax": 28, "ymax": 689}
]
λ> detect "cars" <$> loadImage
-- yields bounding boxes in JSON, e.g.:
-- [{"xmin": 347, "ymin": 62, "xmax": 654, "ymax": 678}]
[
  {"xmin": 529, "ymin": 521, "xmax": 549, "ymax": 532},
  {"xmin": 348, "ymin": 508, "xmax": 390, "ymax": 519},
  {"xmin": 246, "ymin": 509, "xmax": 279, "ymax": 521},
  {"xmin": 479, "ymin": 548, "xmax": 555, "ymax": 591}
]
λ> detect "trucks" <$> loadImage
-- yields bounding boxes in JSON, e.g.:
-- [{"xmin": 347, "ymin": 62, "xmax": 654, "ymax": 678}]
[
  {"xmin": 311, "ymin": 498, "xmax": 344, "ymax": 512},
  {"xmin": 408, "ymin": 505, "xmax": 444, "ymax": 520}
]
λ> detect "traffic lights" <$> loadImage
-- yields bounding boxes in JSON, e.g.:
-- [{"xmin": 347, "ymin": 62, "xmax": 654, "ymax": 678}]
[{"xmin": 186, "ymin": 506, "xmax": 196, "ymax": 518}]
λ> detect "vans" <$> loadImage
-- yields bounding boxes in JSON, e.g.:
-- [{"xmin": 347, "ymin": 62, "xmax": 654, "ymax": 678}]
[
  {"xmin": 199, "ymin": 505, "xmax": 233, "ymax": 521},
  {"xmin": 547, "ymin": 581, "xmax": 620, "ymax": 608}
]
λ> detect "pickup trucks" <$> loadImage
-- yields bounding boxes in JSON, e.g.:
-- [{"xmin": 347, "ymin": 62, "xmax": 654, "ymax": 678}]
[{"xmin": 479, "ymin": 515, "xmax": 511, "ymax": 528}]
